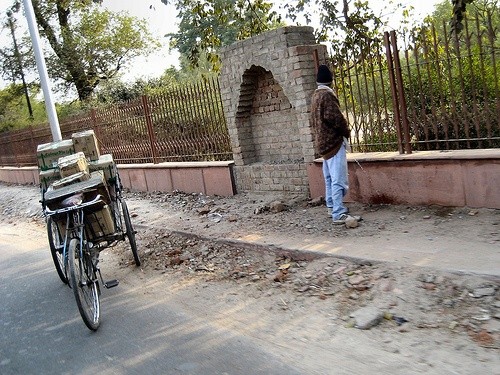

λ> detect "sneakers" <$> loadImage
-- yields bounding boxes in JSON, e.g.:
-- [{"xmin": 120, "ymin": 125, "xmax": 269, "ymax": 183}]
[
  {"xmin": 327, "ymin": 208, "xmax": 333, "ymax": 218},
  {"xmin": 333, "ymin": 214, "xmax": 361, "ymax": 225}
]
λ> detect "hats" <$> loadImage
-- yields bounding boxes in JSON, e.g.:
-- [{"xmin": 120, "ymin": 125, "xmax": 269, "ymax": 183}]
[{"xmin": 316, "ymin": 65, "xmax": 333, "ymax": 86}]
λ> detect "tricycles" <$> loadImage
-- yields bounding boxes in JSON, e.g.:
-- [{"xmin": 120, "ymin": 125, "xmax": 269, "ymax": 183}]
[{"xmin": 38, "ymin": 170, "xmax": 141, "ymax": 331}]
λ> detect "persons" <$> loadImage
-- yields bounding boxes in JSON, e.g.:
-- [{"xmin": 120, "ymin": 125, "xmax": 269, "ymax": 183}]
[{"xmin": 310, "ymin": 65, "xmax": 361, "ymax": 225}]
